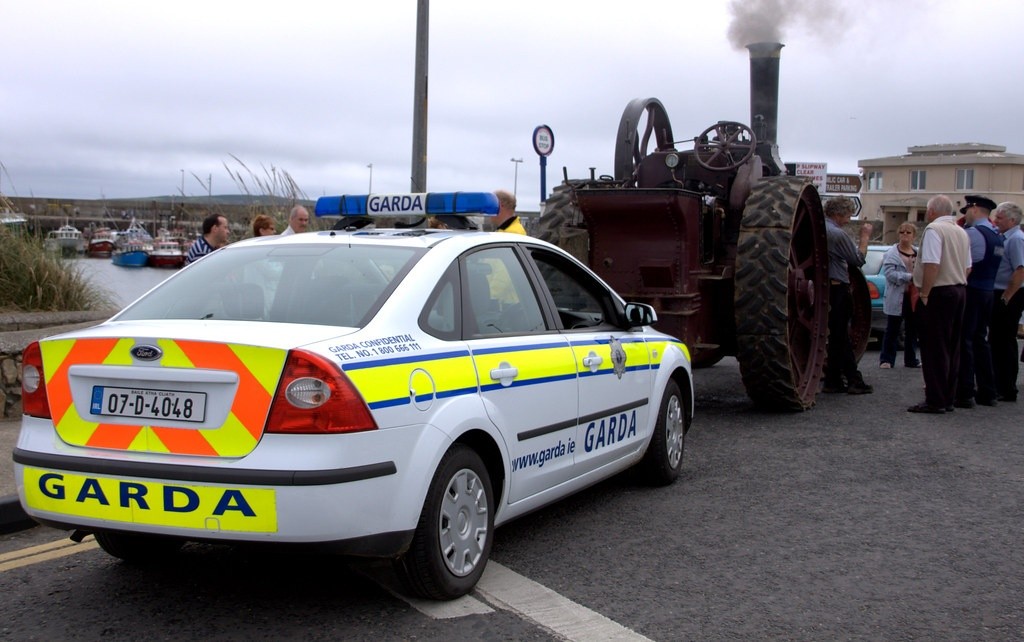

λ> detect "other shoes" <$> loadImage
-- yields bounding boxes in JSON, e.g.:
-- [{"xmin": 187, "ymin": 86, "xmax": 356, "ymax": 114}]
[
  {"xmin": 880, "ymin": 362, "xmax": 891, "ymax": 368},
  {"xmin": 906, "ymin": 391, "xmax": 1018, "ymax": 413},
  {"xmin": 916, "ymin": 362, "xmax": 923, "ymax": 369}
]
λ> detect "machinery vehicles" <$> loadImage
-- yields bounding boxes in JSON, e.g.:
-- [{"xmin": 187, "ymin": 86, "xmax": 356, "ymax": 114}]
[{"xmin": 529, "ymin": 37, "xmax": 877, "ymax": 417}]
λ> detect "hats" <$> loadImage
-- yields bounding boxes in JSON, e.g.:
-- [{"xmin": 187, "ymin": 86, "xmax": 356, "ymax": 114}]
[{"xmin": 959, "ymin": 195, "xmax": 997, "ymax": 214}]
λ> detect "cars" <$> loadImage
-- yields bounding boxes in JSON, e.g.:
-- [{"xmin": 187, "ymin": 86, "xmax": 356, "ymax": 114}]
[
  {"xmin": 850, "ymin": 242, "xmax": 920, "ymax": 352},
  {"xmin": 10, "ymin": 190, "xmax": 699, "ymax": 605}
]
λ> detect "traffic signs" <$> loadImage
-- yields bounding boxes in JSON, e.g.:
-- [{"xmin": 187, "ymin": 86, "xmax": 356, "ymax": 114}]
[
  {"xmin": 825, "ymin": 173, "xmax": 863, "ymax": 194},
  {"xmin": 818, "ymin": 194, "xmax": 862, "ymax": 218}
]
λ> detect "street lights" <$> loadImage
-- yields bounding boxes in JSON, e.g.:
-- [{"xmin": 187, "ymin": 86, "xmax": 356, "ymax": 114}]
[
  {"xmin": 366, "ymin": 163, "xmax": 373, "ymax": 195},
  {"xmin": 510, "ymin": 157, "xmax": 524, "ymax": 197}
]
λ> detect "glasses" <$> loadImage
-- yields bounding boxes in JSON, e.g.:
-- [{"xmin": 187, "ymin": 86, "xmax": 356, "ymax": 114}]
[{"xmin": 899, "ymin": 231, "xmax": 914, "ymax": 234}]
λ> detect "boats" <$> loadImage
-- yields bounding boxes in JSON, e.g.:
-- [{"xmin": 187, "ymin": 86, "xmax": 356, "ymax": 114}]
[
  {"xmin": 0, "ymin": 213, "xmax": 28, "ymax": 228},
  {"xmin": 147, "ymin": 226, "xmax": 184, "ymax": 270},
  {"xmin": 47, "ymin": 224, "xmax": 84, "ymax": 250},
  {"xmin": 111, "ymin": 216, "xmax": 153, "ymax": 267},
  {"xmin": 89, "ymin": 226, "xmax": 115, "ymax": 260}
]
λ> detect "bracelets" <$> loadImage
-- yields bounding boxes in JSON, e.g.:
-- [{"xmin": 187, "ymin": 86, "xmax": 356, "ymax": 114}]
[{"xmin": 920, "ymin": 292, "xmax": 927, "ymax": 297}]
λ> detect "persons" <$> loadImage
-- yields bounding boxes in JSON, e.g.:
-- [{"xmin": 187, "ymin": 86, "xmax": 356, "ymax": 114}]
[
  {"xmin": 281, "ymin": 206, "xmax": 308, "ymax": 235},
  {"xmin": 243, "ymin": 215, "xmax": 282, "ymax": 311},
  {"xmin": 987, "ymin": 200, "xmax": 1024, "ymax": 402},
  {"xmin": 428, "ymin": 214, "xmax": 490, "ymax": 310},
  {"xmin": 907, "ymin": 194, "xmax": 972, "ymax": 413},
  {"xmin": 186, "ymin": 213, "xmax": 229, "ymax": 265},
  {"xmin": 954, "ymin": 196, "xmax": 1004, "ymax": 409},
  {"xmin": 482, "ymin": 189, "xmax": 528, "ymax": 302},
  {"xmin": 824, "ymin": 195, "xmax": 873, "ymax": 394},
  {"xmin": 879, "ymin": 221, "xmax": 922, "ymax": 368}
]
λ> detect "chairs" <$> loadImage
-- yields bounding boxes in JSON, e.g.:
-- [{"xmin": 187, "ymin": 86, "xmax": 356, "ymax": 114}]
[{"xmin": 221, "ymin": 272, "xmax": 492, "ymax": 334}]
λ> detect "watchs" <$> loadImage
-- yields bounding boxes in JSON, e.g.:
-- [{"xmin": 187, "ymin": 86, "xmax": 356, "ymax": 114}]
[{"xmin": 1001, "ymin": 296, "xmax": 1008, "ymax": 304}]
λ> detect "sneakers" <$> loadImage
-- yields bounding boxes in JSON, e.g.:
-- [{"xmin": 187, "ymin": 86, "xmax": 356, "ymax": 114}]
[
  {"xmin": 822, "ymin": 376, "xmax": 848, "ymax": 394},
  {"xmin": 848, "ymin": 371, "xmax": 873, "ymax": 394}
]
